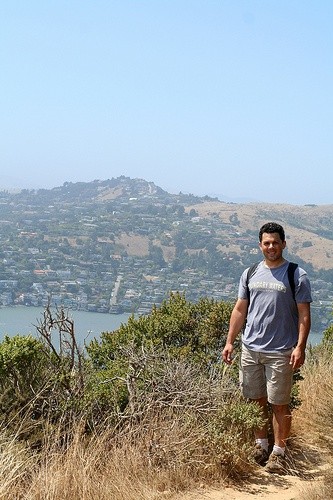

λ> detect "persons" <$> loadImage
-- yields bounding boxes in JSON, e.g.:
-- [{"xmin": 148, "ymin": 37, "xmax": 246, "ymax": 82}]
[{"xmin": 221, "ymin": 223, "xmax": 313, "ymax": 468}]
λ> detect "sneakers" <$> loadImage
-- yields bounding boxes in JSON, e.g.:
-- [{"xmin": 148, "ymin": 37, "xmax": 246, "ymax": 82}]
[
  {"xmin": 249, "ymin": 442, "xmax": 273, "ymax": 466},
  {"xmin": 264, "ymin": 452, "xmax": 286, "ymax": 474}
]
